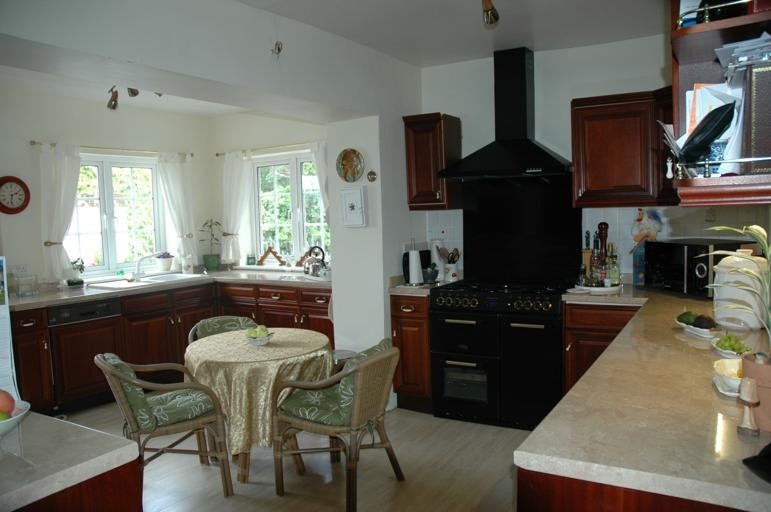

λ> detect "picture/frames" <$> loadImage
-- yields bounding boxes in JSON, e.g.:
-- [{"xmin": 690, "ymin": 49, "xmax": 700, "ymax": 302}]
[
  {"xmin": 510, "ymin": 294, "xmax": 771, "ymax": 512},
  {"xmin": 0, "ymin": 411, "xmax": 147, "ymax": 511}
]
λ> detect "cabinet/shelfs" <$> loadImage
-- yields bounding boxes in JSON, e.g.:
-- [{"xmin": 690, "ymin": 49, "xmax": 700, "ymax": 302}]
[
  {"xmin": 589, "ymin": 238, "xmax": 620, "ymax": 287},
  {"xmin": 319, "ymin": 265, "xmax": 330, "ymax": 279},
  {"xmin": 309, "ymin": 261, "xmax": 321, "ymax": 276}
]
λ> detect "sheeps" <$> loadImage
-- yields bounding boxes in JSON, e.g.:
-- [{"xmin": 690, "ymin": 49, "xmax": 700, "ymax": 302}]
[{"xmin": 135, "ymin": 251, "xmax": 164, "ymax": 276}]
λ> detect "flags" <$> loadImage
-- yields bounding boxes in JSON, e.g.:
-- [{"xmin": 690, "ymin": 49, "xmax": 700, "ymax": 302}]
[{"xmin": 154, "ymin": 255, "xmax": 177, "ymax": 273}]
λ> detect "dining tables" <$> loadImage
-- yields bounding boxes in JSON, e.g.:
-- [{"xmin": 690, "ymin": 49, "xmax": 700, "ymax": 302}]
[
  {"xmin": 197, "ymin": 217, "xmax": 224, "ymax": 273},
  {"xmin": 69, "ymin": 257, "xmax": 86, "ymax": 283}
]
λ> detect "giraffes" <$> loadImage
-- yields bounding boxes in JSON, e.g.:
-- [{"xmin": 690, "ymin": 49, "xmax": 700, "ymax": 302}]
[{"xmin": 429, "ymin": 278, "xmax": 573, "ymax": 315}]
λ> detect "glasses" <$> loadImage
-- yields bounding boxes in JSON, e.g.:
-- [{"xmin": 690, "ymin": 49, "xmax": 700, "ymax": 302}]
[
  {"xmin": 106, "ymin": 85, "xmax": 165, "ymax": 111},
  {"xmin": 481, "ymin": 0, "xmax": 502, "ymax": 26}
]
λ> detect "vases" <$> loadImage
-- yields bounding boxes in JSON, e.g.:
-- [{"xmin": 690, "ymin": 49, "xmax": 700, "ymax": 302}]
[{"xmin": 156, "ymin": 251, "xmax": 172, "ymax": 259}]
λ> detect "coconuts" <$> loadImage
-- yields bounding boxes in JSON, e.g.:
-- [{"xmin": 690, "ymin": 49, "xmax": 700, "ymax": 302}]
[{"xmin": 677, "ymin": 311, "xmax": 696, "ymax": 324}]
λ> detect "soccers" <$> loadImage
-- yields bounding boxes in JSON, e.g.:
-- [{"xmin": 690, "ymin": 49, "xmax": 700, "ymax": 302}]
[
  {"xmin": 0, "ymin": 390, "xmax": 14, "ymax": 413},
  {"xmin": 246, "ymin": 325, "xmax": 268, "ymax": 338},
  {"xmin": 0, "ymin": 412, "xmax": 9, "ymax": 421}
]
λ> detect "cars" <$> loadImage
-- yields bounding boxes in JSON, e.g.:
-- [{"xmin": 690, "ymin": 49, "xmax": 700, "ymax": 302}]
[
  {"xmin": 444, "ymin": 263, "xmax": 458, "ymax": 282},
  {"xmin": 699, "ymin": 139, "xmax": 730, "ymax": 178},
  {"xmin": 247, "ymin": 253, "xmax": 256, "ymax": 264}
]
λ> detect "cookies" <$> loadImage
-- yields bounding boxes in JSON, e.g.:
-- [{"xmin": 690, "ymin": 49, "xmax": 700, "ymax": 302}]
[{"xmin": 642, "ymin": 232, "xmax": 764, "ymax": 300}]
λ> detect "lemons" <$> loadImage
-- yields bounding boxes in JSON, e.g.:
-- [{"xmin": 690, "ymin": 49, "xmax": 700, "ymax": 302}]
[{"xmin": 305, "ymin": 245, "xmax": 327, "ymax": 273}]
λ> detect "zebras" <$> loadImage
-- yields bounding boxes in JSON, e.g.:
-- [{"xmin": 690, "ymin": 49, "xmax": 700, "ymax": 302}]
[{"xmin": 426, "ymin": 310, "xmax": 566, "ymax": 432}]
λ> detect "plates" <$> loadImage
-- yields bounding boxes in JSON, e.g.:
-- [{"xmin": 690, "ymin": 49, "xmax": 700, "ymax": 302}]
[
  {"xmin": 43, "ymin": 293, "xmax": 128, "ymax": 416},
  {"xmin": 569, "ymin": 83, "xmax": 680, "ymax": 211},
  {"xmin": 662, "ymin": 0, "xmax": 771, "ymax": 211},
  {"xmin": 7, "ymin": 301, "xmax": 58, "ymax": 419},
  {"xmin": 558, "ymin": 297, "xmax": 648, "ymax": 401},
  {"xmin": 389, "ymin": 283, "xmax": 433, "ymax": 411},
  {"xmin": 119, "ymin": 278, "xmax": 217, "ymax": 395},
  {"xmin": 256, "ymin": 277, "xmax": 335, "ymax": 353},
  {"xmin": 215, "ymin": 280, "xmax": 261, "ymax": 326},
  {"xmin": 400, "ymin": 111, "xmax": 466, "ymax": 214}
]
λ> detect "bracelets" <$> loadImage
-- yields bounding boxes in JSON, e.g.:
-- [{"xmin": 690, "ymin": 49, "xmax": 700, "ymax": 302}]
[
  {"xmin": 711, "ymin": 358, "xmax": 744, "ymax": 391},
  {"xmin": 420, "ymin": 270, "xmax": 440, "ymax": 282},
  {"xmin": 248, "ymin": 332, "xmax": 276, "ymax": 346},
  {"xmin": 0, "ymin": 399, "xmax": 31, "ymax": 439}
]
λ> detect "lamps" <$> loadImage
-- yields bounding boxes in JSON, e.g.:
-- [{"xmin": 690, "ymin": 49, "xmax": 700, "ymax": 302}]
[
  {"xmin": 268, "ymin": 335, "xmax": 407, "ymax": 512},
  {"xmin": 185, "ymin": 313, "xmax": 309, "ymax": 484},
  {"xmin": 92, "ymin": 348, "xmax": 236, "ymax": 502}
]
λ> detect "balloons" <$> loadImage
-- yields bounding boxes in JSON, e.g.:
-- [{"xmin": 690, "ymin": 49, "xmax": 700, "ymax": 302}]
[{"xmin": 0, "ymin": 175, "xmax": 32, "ymax": 215}]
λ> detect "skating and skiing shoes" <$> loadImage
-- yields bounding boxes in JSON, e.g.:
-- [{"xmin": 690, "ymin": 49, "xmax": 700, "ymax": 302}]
[{"xmin": 717, "ymin": 334, "xmax": 750, "ymax": 354}]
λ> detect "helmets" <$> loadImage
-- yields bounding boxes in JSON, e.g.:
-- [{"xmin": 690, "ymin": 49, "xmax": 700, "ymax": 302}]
[
  {"xmin": 336, "ymin": 148, "xmax": 366, "ymax": 184},
  {"xmin": 675, "ymin": 315, "xmax": 724, "ymax": 339},
  {"xmin": 672, "ymin": 331, "xmax": 713, "ymax": 350},
  {"xmin": 576, "ymin": 284, "xmax": 623, "ymax": 294},
  {"xmin": 712, "ymin": 339, "xmax": 752, "ymax": 359},
  {"xmin": 712, "ymin": 376, "xmax": 741, "ymax": 398}
]
